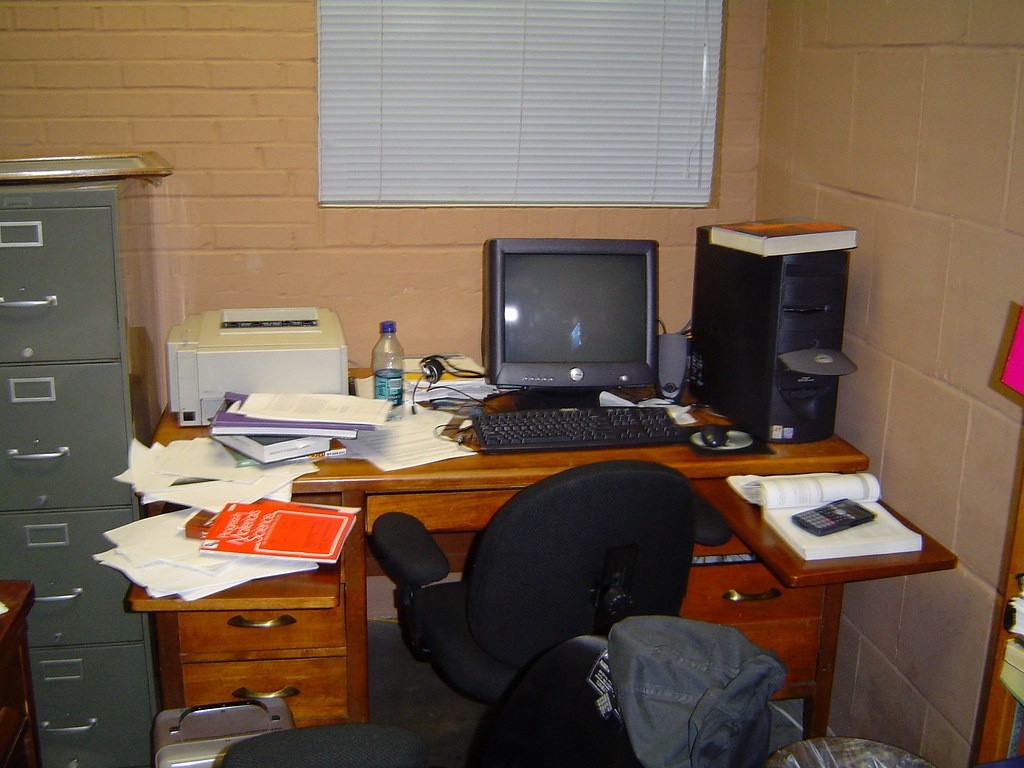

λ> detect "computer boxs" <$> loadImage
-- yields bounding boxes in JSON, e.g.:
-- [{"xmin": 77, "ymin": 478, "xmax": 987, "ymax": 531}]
[{"xmin": 689, "ymin": 226, "xmax": 859, "ymax": 443}]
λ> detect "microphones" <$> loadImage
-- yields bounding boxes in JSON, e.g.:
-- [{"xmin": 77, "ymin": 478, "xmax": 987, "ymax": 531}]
[{"xmin": 411, "ymin": 374, "xmax": 426, "ymax": 414}]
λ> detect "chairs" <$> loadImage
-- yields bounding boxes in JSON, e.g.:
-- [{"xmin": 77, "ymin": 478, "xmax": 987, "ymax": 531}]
[{"xmin": 373, "ymin": 459, "xmax": 735, "ymax": 768}]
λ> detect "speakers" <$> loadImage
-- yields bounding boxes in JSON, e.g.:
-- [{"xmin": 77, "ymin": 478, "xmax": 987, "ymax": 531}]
[{"xmin": 655, "ymin": 334, "xmax": 689, "ymax": 407}]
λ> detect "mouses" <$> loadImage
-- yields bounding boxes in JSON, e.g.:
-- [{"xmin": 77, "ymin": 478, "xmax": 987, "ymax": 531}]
[{"xmin": 702, "ymin": 424, "xmax": 727, "ymax": 447}]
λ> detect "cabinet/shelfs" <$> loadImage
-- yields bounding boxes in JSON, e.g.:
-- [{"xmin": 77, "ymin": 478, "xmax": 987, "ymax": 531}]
[{"xmin": 0, "ymin": 177, "xmax": 160, "ymax": 768}]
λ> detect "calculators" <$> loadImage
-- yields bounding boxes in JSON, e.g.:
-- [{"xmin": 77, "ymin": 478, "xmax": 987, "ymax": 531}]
[{"xmin": 792, "ymin": 497, "xmax": 876, "ymax": 535}]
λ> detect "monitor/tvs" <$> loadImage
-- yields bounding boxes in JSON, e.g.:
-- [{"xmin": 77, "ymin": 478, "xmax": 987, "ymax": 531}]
[{"xmin": 479, "ymin": 238, "xmax": 660, "ymax": 409}]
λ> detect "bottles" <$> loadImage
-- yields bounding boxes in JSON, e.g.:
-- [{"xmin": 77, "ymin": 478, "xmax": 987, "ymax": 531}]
[{"xmin": 372, "ymin": 321, "xmax": 405, "ymax": 422}]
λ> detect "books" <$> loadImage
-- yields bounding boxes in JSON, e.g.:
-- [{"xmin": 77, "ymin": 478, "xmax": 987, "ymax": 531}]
[
  {"xmin": 184, "ymin": 497, "xmax": 362, "ymax": 563},
  {"xmin": 222, "ymin": 306, "xmax": 323, "ymax": 333},
  {"xmin": 725, "ymin": 473, "xmax": 921, "ymax": 562},
  {"xmin": 208, "ymin": 392, "xmax": 393, "ymax": 469},
  {"xmin": 709, "ymin": 216, "xmax": 859, "ymax": 256}
]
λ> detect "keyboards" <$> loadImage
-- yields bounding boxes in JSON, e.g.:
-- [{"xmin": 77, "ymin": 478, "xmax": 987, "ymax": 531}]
[{"xmin": 470, "ymin": 406, "xmax": 690, "ymax": 453}]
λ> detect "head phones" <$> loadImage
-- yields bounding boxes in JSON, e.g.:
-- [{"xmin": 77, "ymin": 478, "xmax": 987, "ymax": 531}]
[{"xmin": 419, "ymin": 354, "xmax": 485, "ymax": 383}]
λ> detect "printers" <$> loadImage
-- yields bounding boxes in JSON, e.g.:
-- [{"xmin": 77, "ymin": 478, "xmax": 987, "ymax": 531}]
[{"xmin": 166, "ymin": 307, "xmax": 349, "ymax": 426}]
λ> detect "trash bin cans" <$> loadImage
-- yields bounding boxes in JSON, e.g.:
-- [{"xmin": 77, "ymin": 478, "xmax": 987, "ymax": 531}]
[{"xmin": 765, "ymin": 735, "xmax": 940, "ymax": 768}]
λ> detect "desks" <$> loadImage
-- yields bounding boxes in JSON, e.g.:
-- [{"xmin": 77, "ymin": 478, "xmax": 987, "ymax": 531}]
[{"xmin": 124, "ymin": 367, "xmax": 959, "ymax": 740}]
